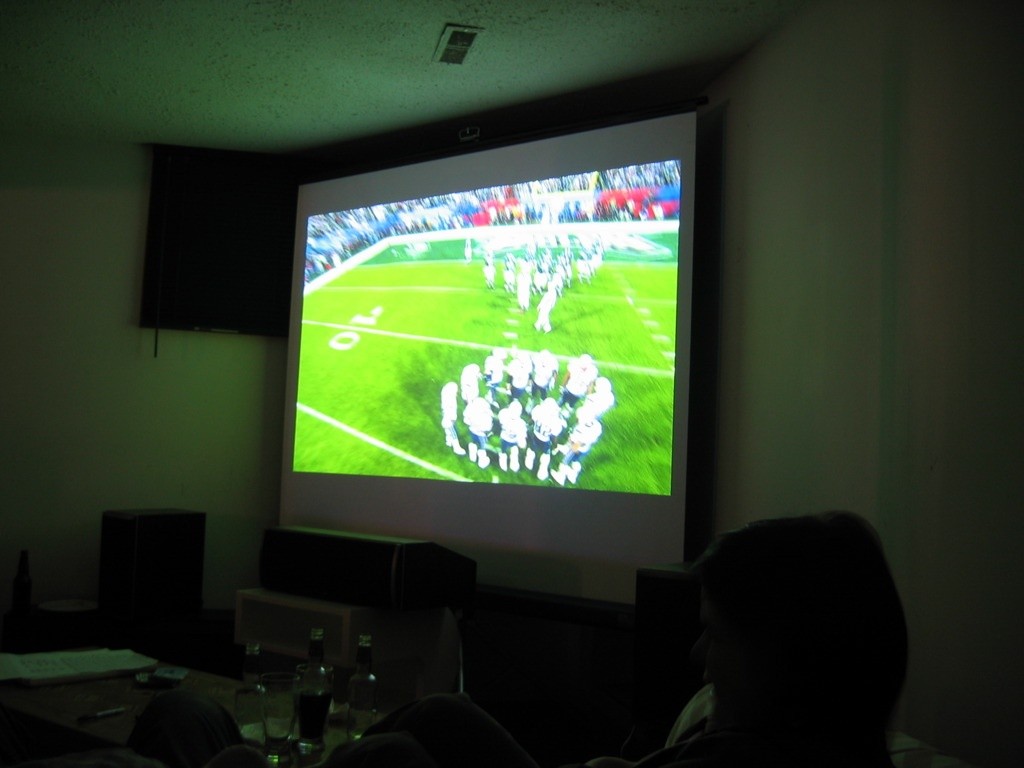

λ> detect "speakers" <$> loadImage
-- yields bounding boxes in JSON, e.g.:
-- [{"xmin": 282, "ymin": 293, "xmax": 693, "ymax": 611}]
[{"xmin": 98, "ymin": 509, "xmax": 205, "ymax": 610}]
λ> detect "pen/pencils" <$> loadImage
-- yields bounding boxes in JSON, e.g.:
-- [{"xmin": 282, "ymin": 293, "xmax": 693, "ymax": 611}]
[{"xmin": 79, "ymin": 707, "xmax": 128, "ymax": 719}]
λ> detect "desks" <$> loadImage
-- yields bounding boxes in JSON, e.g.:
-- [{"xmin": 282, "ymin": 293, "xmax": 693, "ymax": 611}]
[
  {"xmin": 0, "ymin": 646, "xmax": 389, "ymax": 768},
  {"xmin": 234, "ymin": 583, "xmax": 474, "ymax": 696}
]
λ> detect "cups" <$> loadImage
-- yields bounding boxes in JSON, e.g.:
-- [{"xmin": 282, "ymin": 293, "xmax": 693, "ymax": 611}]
[
  {"xmin": 295, "ymin": 664, "xmax": 336, "ymax": 753},
  {"xmin": 259, "ymin": 672, "xmax": 301, "ymax": 764}
]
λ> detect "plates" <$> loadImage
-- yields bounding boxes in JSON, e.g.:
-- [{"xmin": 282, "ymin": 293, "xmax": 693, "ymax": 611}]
[{"xmin": 39, "ymin": 599, "xmax": 99, "ymax": 612}]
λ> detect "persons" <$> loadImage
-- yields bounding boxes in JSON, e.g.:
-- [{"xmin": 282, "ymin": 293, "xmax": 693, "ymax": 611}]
[
  {"xmin": 439, "ymin": 349, "xmax": 614, "ymax": 487},
  {"xmin": 399, "ymin": 170, "xmax": 609, "ymax": 235},
  {"xmin": 604, "ymin": 160, "xmax": 681, "ymax": 223},
  {"xmin": 122, "ymin": 691, "xmax": 242, "ymax": 768},
  {"xmin": 303, "ymin": 202, "xmax": 400, "ymax": 289},
  {"xmin": 200, "ymin": 511, "xmax": 914, "ymax": 768},
  {"xmin": 483, "ymin": 244, "xmax": 608, "ymax": 335}
]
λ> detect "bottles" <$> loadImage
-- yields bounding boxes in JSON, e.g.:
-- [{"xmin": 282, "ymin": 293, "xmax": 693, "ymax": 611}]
[
  {"xmin": 347, "ymin": 633, "xmax": 377, "ymax": 741},
  {"xmin": 296, "ymin": 628, "xmax": 331, "ymax": 731},
  {"xmin": 11, "ymin": 549, "xmax": 32, "ymax": 623}
]
